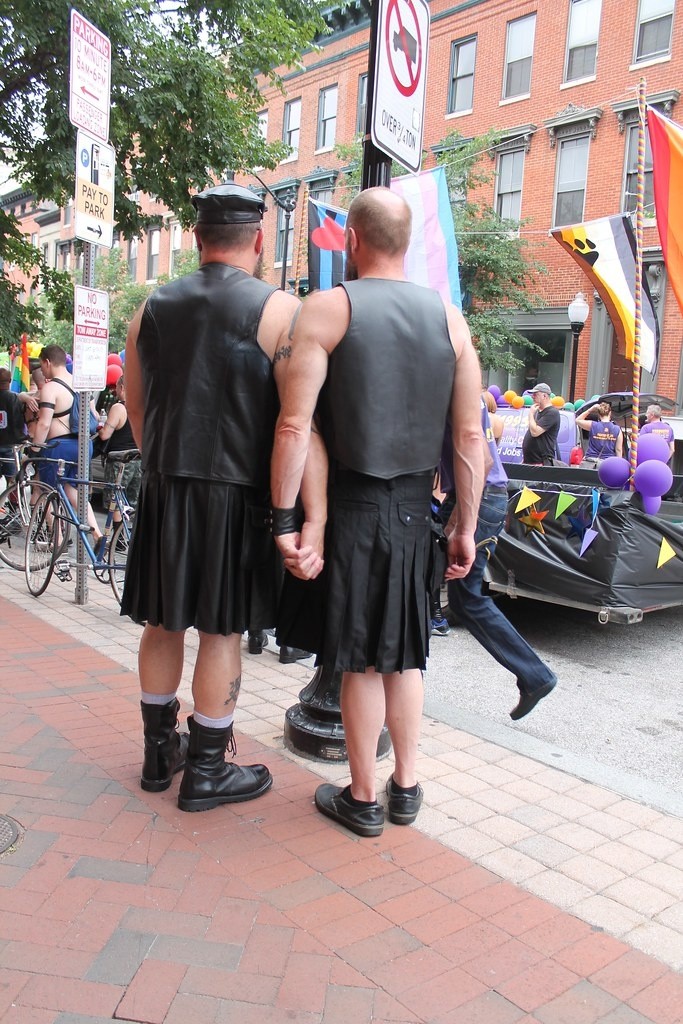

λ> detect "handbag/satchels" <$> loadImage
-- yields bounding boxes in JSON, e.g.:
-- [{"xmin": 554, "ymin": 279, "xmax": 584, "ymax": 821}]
[
  {"xmin": 579, "ymin": 459, "xmax": 597, "ymax": 469},
  {"xmin": 544, "ymin": 457, "xmax": 569, "ymax": 468}
]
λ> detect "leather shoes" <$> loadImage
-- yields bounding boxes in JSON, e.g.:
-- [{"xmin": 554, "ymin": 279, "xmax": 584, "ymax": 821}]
[
  {"xmin": 315, "ymin": 783, "xmax": 384, "ymax": 836},
  {"xmin": 279, "ymin": 645, "xmax": 312, "ymax": 663},
  {"xmin": 248, "ymin": 632, "xmax": 268, "ymax": 654},
  {"xmin": 386, "ymin": 772, "xmax": 424, "ymax": 823}
]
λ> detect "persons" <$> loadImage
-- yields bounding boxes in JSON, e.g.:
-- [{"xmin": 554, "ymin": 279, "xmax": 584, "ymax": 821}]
[
  {"xmin": 248, "ymin": 630, "xmax": 313, "ymax": 664},
  {"xmin": 0, "ymin": 344, "xmax": 109, "ymax": 559},
  {"xmin": 120, "ymin": 183, "xmax": 330, "ymax": 811},
  {"xmin": 431, "ymin": 397, "xmax": 558, "ymax": 720},
  {"xmin": 640, "ymin": 404, "xmax": 675, "ymax": 463},
  {"xmin": 521, "ymin": 383, "xmax": 560, "ymax": 466},
  {"xmin": 97, "ymin": 375, "xmax": 143, "ymax": 552},
  {"xmin": 574, "ymin": 402, "xmax": 623, "ymax": 470},
  {"xmin": 268, "ymin": 186, "xmax": 486, "ymax": 838},
  {"xmin": 482, "ymin": 391, "xmax": 504, "ymax": 446}
]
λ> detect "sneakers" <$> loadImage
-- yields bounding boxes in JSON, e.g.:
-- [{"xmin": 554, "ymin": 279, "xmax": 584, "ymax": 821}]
[
  {"xmin": 510, "ymin": 677, "xmax": 557, "ymax": 720},
  {"xmin": 430, "ymin": 618, "xmax": 450, "ymax": 635}
]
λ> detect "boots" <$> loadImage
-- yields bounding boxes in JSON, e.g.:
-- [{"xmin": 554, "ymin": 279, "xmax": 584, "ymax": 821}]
[
  {"xmin": 178, "ymin": 714, "xmax": 273, "ymax": 812},
  {"xmin": 141, "ymin": 698, "xmax": 189, "ymax": 792}
]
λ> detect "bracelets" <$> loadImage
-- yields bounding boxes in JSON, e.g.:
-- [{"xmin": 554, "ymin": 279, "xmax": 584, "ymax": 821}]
[
  {"xmin": 264, "ymin": 506, "xmax": 300, "ymax": 536},
  {"xmin": 588, "ymin": 409, "xmax": 590, "ymax": 413}
]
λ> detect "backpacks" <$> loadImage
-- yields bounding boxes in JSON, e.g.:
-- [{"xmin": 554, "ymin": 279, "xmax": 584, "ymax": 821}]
[{"xmin": 51, "ymin": 377, "xmax": 98, "ymax": 435}]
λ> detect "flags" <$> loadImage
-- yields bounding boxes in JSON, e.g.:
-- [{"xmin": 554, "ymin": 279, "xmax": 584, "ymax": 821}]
[
  {"xmin": 647, "ymin": 105, "xmax": 683, "ymax": 319},
  {"xmin": 306, "ymin": 166, "xmax": 464, "ymax": 314},
  {"xmin": 7, "ymin": 333, "xmax": 29, "ymax": 440},
  {"xmin": 550, "ymin": 212, "xmax": 661, "ymax": 382}
]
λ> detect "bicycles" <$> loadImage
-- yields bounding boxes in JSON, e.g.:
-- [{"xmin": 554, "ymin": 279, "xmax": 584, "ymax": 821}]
[
  {"xmin": 16, "ymin": 448, "xmax": 158, "ymax": 627},
  {"xmin": 0, "ymin": 439, "xmax": 72, "ymax": 571}
]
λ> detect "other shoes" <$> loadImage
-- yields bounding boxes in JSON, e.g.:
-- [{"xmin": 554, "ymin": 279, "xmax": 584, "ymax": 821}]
[
  {"xmin": 43, "ymin": 546, "xmax": 68, "ymax": 553},
  {"xmin": 113, "ymin": 540, "xmax": 126, "ymax": 551},
  {"xmin": 94, "ymin": 536, "xmax": 110, "ymax": 556}
]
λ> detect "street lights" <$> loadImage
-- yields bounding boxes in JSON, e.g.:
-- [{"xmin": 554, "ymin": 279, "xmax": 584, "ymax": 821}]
[{"xmin": 567, "ymin": 293, "xmax": 591, "ymax": 404}]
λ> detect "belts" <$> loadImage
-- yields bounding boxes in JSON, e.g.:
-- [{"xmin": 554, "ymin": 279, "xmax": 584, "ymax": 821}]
[{"xmin": 488, "ymin": 485, "xmax": 507, "ymax": 493}]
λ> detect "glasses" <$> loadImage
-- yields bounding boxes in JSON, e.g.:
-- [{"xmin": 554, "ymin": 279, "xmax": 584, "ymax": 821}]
[{"xmin": 41, "ymin": 347, "xmax": 50, "ymax": 362}]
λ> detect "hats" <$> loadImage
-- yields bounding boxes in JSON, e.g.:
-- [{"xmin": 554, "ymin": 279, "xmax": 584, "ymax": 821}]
[
  {"xmin": 527, "ymin": 383, "xmax": 552, "ymax": 395},
  {"xmin": 191, "ymin": 184, "xmax": 268, "ymax": 224}
]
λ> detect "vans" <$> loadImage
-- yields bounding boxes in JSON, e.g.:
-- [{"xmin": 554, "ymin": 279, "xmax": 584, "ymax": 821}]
[{"xmin": 486, "ymin": 390, "xmax": 680, "ymax": 468}]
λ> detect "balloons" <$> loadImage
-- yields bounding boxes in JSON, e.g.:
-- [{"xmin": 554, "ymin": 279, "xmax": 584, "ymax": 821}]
[
  {"xmin": 633, "ymin": 459, "xmax": 673, "ymax": 498},
  {"xmin": 106, "ymin": 349, "xmax": 127, "ymax": 386},
  {"xmin": 488, "ymin": 385, "xmax": 602, "ymax": 413},
  {"xmin": 598, "ymin": 456, "xmax": 630, "ymax": 490},
  {"xmin": 636, "ymin": 433, "xmax": 671, "ymax": 464},
  {"xmin": 642, "ymin": 495, "xmax": 662, "ymax": 515}
]
